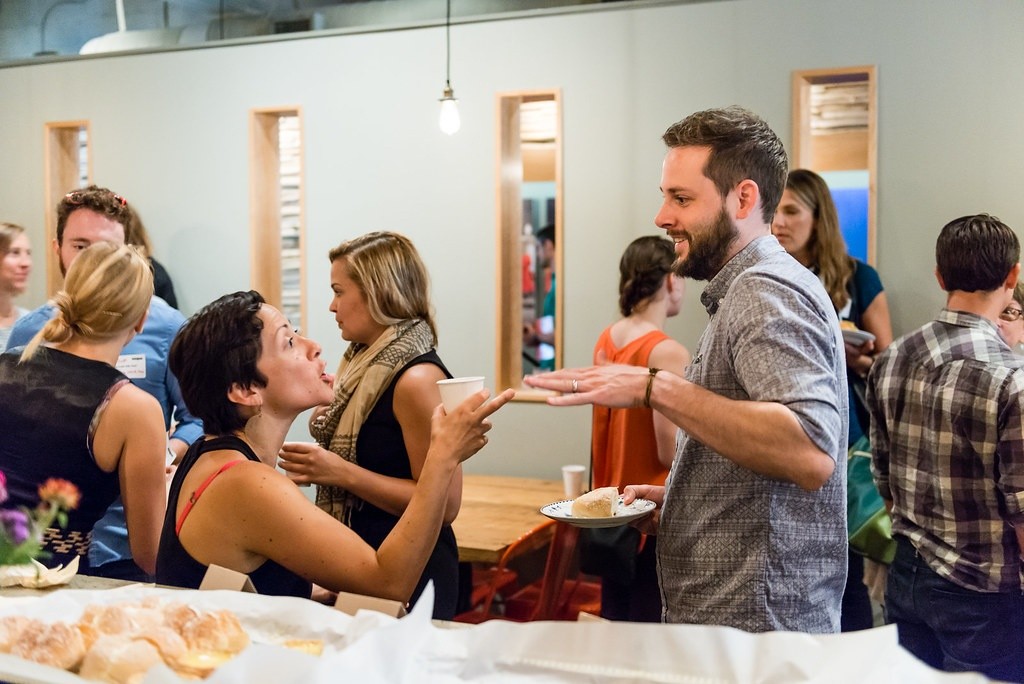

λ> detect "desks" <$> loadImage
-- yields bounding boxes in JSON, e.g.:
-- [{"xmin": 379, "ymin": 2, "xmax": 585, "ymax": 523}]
[{"xmin": 449, "ymin": 473, "xmax": 595, "ymax": 562}]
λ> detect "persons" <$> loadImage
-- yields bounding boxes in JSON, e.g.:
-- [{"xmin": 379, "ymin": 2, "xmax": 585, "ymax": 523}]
[
  {"xmin": 1, "ymin": 186, "xmax": 204, "ymax": 583},
  {"xmin": 997, "ymin": 281, "xmax": 1024, "ymax": 352},
  {"xmin": 772, "ymin": 167, "xmax": 896, "ymax": 634},
  {"xmin": 155, "ymin": 289, "xmax": 515, "ymax": 607},
  {"xmin": 277, "ymin": 231, "xmax": 462, "ymax": 619},
  {"xmin": 520, "ymin": 107, "xmax": 850, "ymax": 633},
  {"xmin": 864, "ymin": 212, "xmax": 1023, "ymax": 684},
  {"xmin": 590, "ymin": 236, "xmax": 690, "ymax": 522},
  {"xmin": 523, "ymin": 222, "xmax": 555, "ymax": 375}
]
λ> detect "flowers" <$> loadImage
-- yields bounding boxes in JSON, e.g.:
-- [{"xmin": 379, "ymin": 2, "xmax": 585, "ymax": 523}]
[{"xmin": 0, "ymin": 469, "xmax": 80, "ymax": 591}]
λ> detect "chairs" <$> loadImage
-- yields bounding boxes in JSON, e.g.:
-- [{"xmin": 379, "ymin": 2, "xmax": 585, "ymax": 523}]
[{"xmin": 460, "ymin": 520, "xmax": 601, "ymax": 624}]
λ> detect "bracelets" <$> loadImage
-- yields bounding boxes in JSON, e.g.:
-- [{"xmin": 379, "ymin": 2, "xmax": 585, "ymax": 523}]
[{"xmin": 643, "ymin": 366, "xmax": 661, "ymax": 408}]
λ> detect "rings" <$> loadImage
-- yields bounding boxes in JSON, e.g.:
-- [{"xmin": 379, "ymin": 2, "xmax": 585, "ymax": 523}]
[{"xmin": 572, "ymin": 379, "xmax": 578, "ymax": 392}]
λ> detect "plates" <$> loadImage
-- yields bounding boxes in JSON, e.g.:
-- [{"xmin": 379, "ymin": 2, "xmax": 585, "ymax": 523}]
[
  {"xmin": 841, "ymin": 329, "xmax": 875, "ymax": 346},
  {"xmin": 540, "ymin": 497, "xmax": 657, "ymax": 528}
]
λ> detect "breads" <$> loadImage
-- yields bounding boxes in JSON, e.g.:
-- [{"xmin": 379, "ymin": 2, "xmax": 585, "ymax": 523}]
[
  {"xmin": 572, "ymin": 486, "xmax": 619, "ymax": 517},
  {"xmin": 0, "ymin": 596, "xmax": 324, "ymax": 684},
  {"xmin": 839, "ymin": 320, "xmax": 858, "ymax": 332}
]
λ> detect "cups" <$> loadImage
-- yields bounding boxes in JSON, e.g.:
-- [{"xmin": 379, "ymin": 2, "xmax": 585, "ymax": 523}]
[
  {"xmin": 562, "ymin": 464, "xmax": 586, "ymax": 500},
  {"xmin": 436, "ymin": 377, "xmax": 485, "ymax": 416},
  {"xmin": 284, "ymin": 442, "xmax": 319, "ymax": 488}
]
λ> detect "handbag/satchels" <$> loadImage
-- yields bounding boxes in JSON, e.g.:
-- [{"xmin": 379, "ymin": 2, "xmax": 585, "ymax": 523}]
[
  {"xmin": 849, "ymin": 432, "xmax": 899, "ymax": 570},
  {"xmin": 576, "ymin": 526, "xmax": 600, "ymax": 579}
]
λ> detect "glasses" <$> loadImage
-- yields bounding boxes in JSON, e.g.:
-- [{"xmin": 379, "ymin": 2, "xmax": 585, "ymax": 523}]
[
  {"xmin": 996, "ymin": 305, "xmax": 1024, "ymax": 323},
  {"xmin": 65, "ymin": 189, "xmax": 128, "ymax": 210}
]
